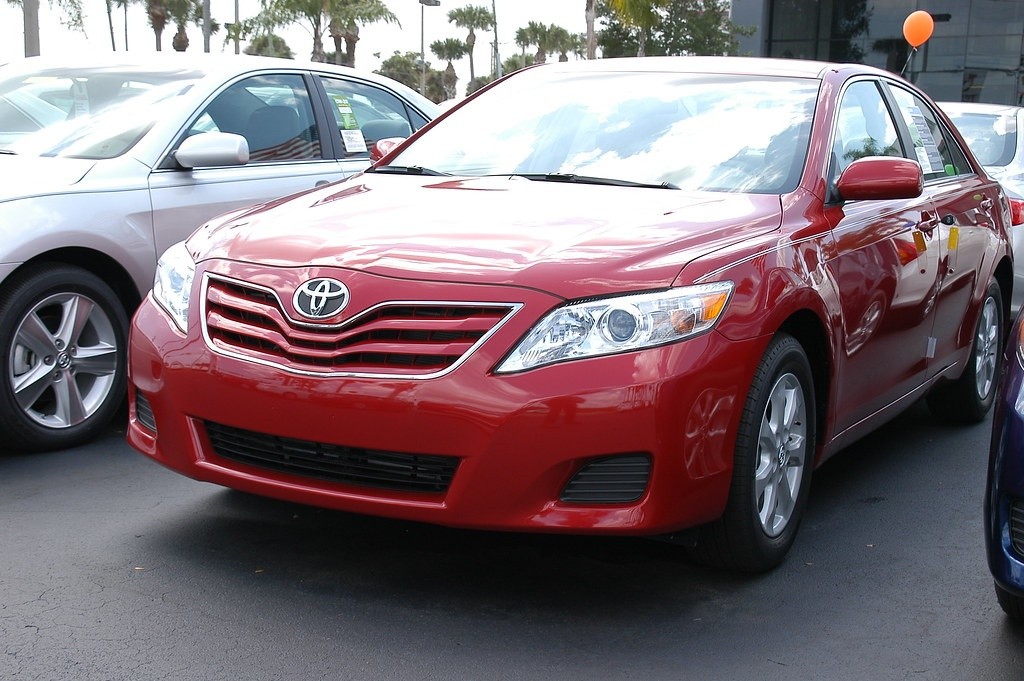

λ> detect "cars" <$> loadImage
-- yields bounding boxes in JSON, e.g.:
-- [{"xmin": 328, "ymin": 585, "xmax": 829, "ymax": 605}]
[
  {"xmin": 119, "ymin": 50, "xmax": 1010, "ymax": 586},
  {"xmin": 923, "ymin": 98, "xmax": 1022, "ymax": 321},
  {"xmin": 2, "ymin": 54, "xmax": 436, "ymax": 464}
]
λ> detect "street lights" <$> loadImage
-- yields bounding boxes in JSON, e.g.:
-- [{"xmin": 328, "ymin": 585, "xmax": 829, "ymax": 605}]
[{"xmin": 419, "ymin": 0, "xmax": 440, "ymax": 97}]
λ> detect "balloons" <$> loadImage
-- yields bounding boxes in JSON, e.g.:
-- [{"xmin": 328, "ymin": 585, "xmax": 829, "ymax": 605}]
[{"xmin": 903, "ymin": 10, "xmax": 934, "ymax": 47}]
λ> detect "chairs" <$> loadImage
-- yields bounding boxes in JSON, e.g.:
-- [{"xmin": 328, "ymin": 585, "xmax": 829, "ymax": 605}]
[{"xmin": 244, "ymin": 106, "xmax": 299, "ymax": 154}]
